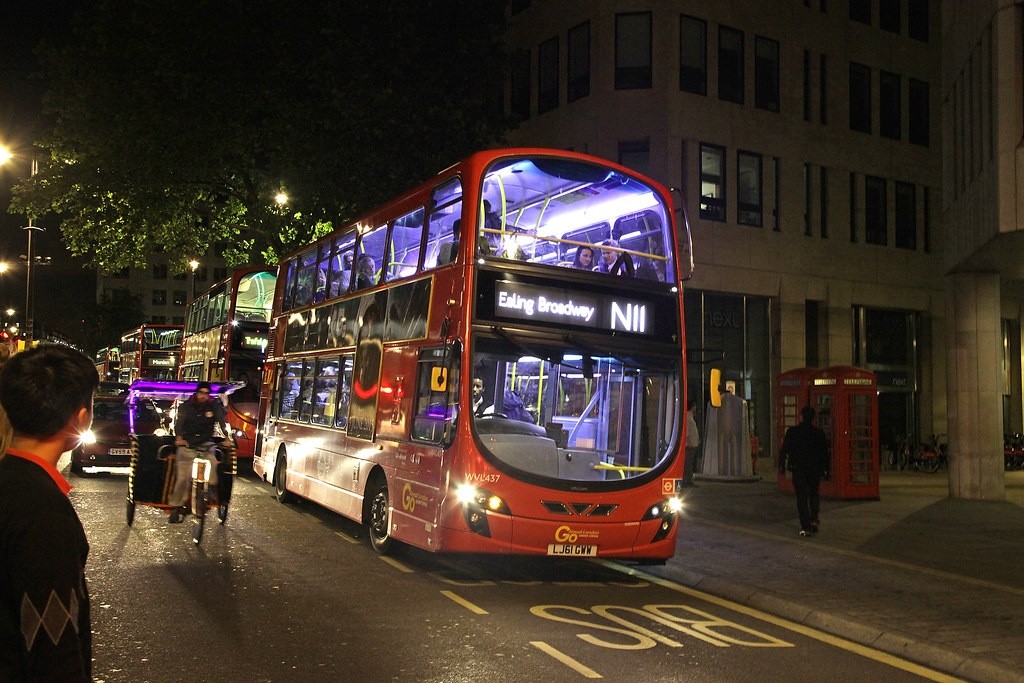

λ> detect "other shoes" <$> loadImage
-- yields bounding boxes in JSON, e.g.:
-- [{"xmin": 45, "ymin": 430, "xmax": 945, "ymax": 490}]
[
  {"xmin": 687, "ymin": 482, "xmax": 699, "ymax": 487},
  {"xmin": 810, "ymin": 522, "xmax": 818, "ymax": 533},
  {"xmin": 799, "ymin": 530, "xmax": 810, "ymax": 536},
  {"xmin": 168, "ymin": 509, "xmax": 179, "ymax": 523}
]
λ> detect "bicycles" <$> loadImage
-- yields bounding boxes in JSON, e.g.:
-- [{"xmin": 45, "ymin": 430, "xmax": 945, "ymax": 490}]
[
  {"xmin": 888, "ymin": 433, "xmax": 948, "ymax": 474},
  {"xmin": 1002, "ymin": 431, "xmax": 1024, "ymax": 471}
]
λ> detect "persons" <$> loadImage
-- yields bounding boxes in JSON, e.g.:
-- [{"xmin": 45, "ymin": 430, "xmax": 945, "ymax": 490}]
[
  {"xmin": 565, "ymin": 239, "xmax": 629, "ymax": 275},
  {"xmin": 0, "ymin": 344, "xmax": 100, "ymax": 683},
  {"xmin": 284, "ymin": 365, "xmax": 350, "ymax": 419},
  {"xmin": 168, "ymin": 382, "xmax": 228, "ymax": 524},
  {"xmin": 635, "ymin": 238, "xmax": 667, "ymax": 282},
  {"xmin": 778, "ymin": 407, "xmax": 832, "ymax": 537},
  {"xmin": 440, "ymin": 199, "xmax": 537, "ymax": 264},
  {"xmin": 287, "ymin": 314, "xmax": 327, "ymax": 350},
  {"xmin": 685, "ymin": 402, "xmax": 700, "ymax": 489},
  {"xmin": 291, "ymin": 248, "xmax": 395, "ymax": 303},
  {"xmin": 473, "ymin": 374, "xmax": 494, "ymax": 420}
]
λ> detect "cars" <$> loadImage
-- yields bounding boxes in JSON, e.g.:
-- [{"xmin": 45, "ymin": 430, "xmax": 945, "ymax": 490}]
[{"xmin": 71, "ymin": 396, "xmax": 133, "ymax": 474}]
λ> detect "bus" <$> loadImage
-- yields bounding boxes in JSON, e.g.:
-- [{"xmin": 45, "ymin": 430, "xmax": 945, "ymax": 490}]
[
  {"xmin": 177, "ymin": 261, "xmax": 280, "ymax": 471},
  {"xmin": 117, "ymin": 324, "xmax": 184, "ymax": 387},
  {"xmin": 251, "ymin": 147, "xmax": 726, "ymax": 568},
  {"xmin": 95, "ymin": 345, "xmax": 121, "ymax": 382}
]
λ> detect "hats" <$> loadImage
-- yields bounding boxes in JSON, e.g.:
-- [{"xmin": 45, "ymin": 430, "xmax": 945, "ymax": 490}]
[{"xmin": 196, "ymin": 382, "xmax": 211, "ymax": 392}]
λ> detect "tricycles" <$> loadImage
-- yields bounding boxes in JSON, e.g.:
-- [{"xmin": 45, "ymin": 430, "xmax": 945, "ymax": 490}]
[{"xmin": 125, "ymin": 379, "xmax": 246, "ymax": 546}]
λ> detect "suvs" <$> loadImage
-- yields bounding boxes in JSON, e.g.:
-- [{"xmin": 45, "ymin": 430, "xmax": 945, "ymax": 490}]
[{"xmin": 92, "ymin": 384, "xmax": 130, "ymax": 397}]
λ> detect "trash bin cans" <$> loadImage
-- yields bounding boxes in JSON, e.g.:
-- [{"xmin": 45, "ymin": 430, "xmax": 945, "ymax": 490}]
[{"xmin": 546, "ymin": 423, "xmax": 569, "ymax": 448}]
